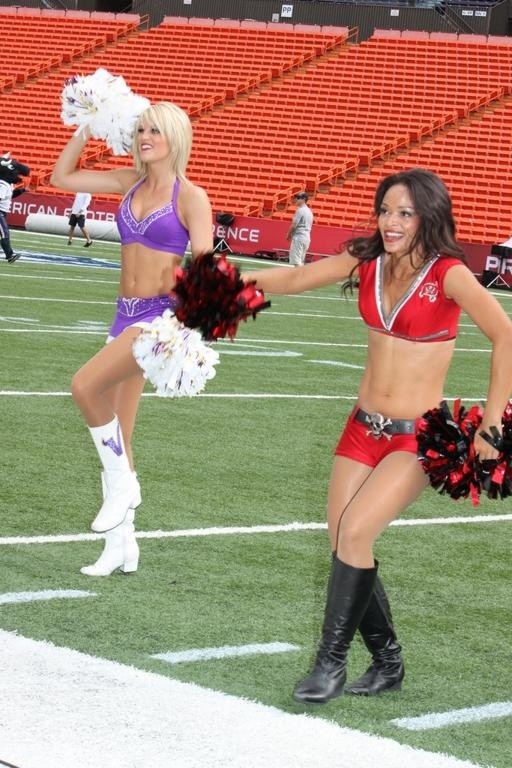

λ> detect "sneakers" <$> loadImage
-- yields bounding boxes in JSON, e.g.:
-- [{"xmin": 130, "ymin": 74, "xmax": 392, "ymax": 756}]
[
  {"xmin": 84, "ymin": 241, "xmax": 92, "ymax": 247},
  {"xmin": 8, "ymin": 254, "xmax": 20, "ymax": 263}
]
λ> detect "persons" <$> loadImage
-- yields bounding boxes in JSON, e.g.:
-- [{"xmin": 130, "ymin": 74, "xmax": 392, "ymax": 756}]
[
  {"xmin": 0, "ymin": 167, "xmax": 31, "ymax": 263},
  {"xmin": 68, "ymin": 192, "xmax": 93, "ymax": 247},
  {"xmin": 50, "ymin": 102, "xmax": 214, "ymax": 577},
  {"xmin": 286, "ymin": 192, "xmax": 313, "ymax": 267},
  {"xmin": 243, "ymin": 169, "xmax": 512, "ymax": 704}
]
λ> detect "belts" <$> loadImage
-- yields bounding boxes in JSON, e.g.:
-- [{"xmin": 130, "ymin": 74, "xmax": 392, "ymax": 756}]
[{"xmin": 355, "ymin": 410, "xmax": 417, "ymax": 436}]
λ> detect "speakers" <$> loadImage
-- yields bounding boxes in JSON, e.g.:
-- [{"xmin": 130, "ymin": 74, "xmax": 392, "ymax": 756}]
[
  {"xmin": 491, "ymin": 245, "xmax": 512, "ymax": 259},
  {"xmin": 216, "ymin": 213, "xmax": 235, "ymax": 226}
]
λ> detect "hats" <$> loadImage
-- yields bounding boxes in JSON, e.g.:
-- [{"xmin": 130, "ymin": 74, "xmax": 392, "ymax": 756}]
[{"xmin": 294, "ymin": 192, "xmax": 308, "ymax": 201}]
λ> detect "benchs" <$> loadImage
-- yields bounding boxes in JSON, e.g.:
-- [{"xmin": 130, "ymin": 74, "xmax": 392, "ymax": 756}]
[
  {"xmin": 271, "ymin": 100, "xmax": 512, "ymax": 248},
  {"xmin": 0, "ymin": 22, "xmax": 346, "ymax": 190},
  {"xmin": 0, "ymin": 15, "xmax": 139, "ymax": 91},
  {"xmin": 33, "ymin": 39, "xmax": 512, "ymax": 219}
]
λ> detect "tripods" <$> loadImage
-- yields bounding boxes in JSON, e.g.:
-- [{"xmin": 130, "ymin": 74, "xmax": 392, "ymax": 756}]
[
  {"xmin": 486, "ymin": 258, "xmax": 512, "ymax": 290},
  {"xmin": 213, "ymin": 224, "xmax": 234, "ymax": 255}
]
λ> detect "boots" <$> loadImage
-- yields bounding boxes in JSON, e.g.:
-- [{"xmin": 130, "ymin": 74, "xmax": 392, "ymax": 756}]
[
  {"xmin": 291, "ymin": 557, "xmax": 377, "ymax": 704},
  {"xmin": 332, "ymin": 550, "xmax": 404, "ymax": 697},
  {"xmin": 88, "ymin": 414, "xmax": 142, "ymax": 532},
  {"xmin": 80, "ymin": 471, "xmax": 140, "ymax": 574}
]
